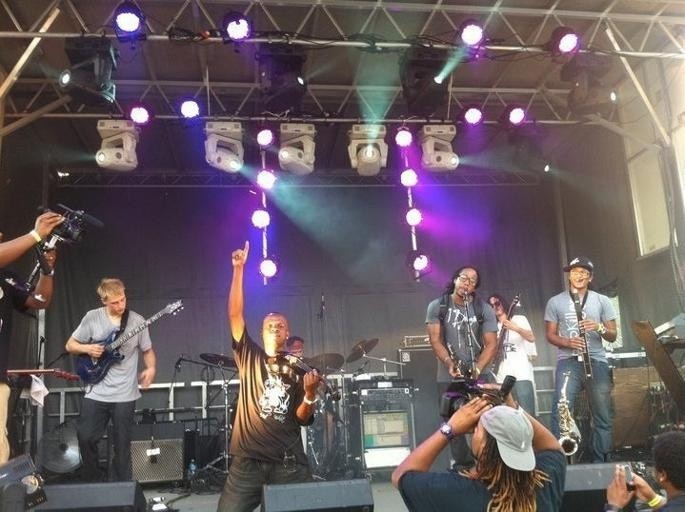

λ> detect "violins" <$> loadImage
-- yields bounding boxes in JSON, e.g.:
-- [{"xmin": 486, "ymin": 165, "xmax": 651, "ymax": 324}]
[{"xmin": 268, "ymin": 353, "xmax": 341, "ymax": 401}]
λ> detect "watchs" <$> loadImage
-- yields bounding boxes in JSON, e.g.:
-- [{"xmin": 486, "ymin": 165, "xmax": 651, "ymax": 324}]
[{"xmin": 440, "ymin": 422, "xmax": 456, "ymax": 441}]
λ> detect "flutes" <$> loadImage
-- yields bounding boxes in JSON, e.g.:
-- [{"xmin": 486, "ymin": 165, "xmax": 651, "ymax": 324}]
[{"xmin": 572, "ymin": 289, "xmax": 594, "ymax": 381}]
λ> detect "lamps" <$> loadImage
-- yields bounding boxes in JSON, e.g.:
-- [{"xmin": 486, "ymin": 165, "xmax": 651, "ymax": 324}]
[
  {"xmin": 278, "ymin": 122, "xmax": 318, "ymax": 176},
  {"xmin": 458, "ymin": 20, "xmax": 484, "ymax": 47},
  {"xmin": 551, "ymin": 25, "xmax": 579, "ymax": 56},
  {"xmin": 221, "ymin": 10, "xmax": 250, "ymax": 42},
  {"xmin": 58, "ymin": 36, "xmax": 117, "ymax": 105},
  {"xmin": 113, "ymin": 3, "xmax": 146, "ymax": 44},
  {"xmin": 251, "ymin": 129, "xmax": 277, "ymax": 286},
  {"xmin": 127, "ymin": 102, "xmax": 154, "ymax": 132},
  {"xmin": 416, "ymin": 123, "xmax": 459, "ymax": 171},
  {"xmin": 398, "ymin": 45, "xmax": 449, "ymax": 114},
  {"xmin": 395, "ymin": 127, "xmax": 428, "ymax": 282},
  {"xmin": 95, "ymin": 119, "xmax": 140, "ymax": 172},
  {"xmin": 345, "ymin": 124, "xmax": 389, "ymax": 177},
  {"xmin": 203, "ymin": 121, "xmax": 244, "ymax": 173},
  {"xmin": 254, "ymin": 43, "xmax": 308, "ymax": 111},
  {"xmin": 177, "ymin": 97, "xmax": 205, "ymax": 130}
]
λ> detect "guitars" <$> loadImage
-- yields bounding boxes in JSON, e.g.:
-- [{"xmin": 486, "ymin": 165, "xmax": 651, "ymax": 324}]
[
  {"xmin": 75, "ymin": 298, "xmax": 184, "ymax": 385},
  {"xmin": 490, "ymin": 294, "xmax": 519, "ymax": 376}
]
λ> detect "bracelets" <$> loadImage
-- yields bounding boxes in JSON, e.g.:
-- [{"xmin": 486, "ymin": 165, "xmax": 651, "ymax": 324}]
[
  {"xmin": 648, "ymin": 494, "xmax": 663, "ymax": 506},
  {"xmin": 303, "ymin": 395, "xmax": 319, "ymax": 406},
  {"xmin": 29, "ymin": 230, "xmax": 43, "ymax": 244},
  {"xmin": 598, "ymin": 323, "xmax": 608, "ymax": 336},
  {"xmin": 444, "ymin": 357, "xmax": 450, "ymax": 366},
  {"xmin": 516, "ymin": 405, "xmax": 524, "ymax": 413},
  {"xmin": 605, "ymin": 502, "xmax": 623, "ymax": 511},
  {"xmin": 475, "ymin": 366, "xmax": 480, "ymax": 377}
]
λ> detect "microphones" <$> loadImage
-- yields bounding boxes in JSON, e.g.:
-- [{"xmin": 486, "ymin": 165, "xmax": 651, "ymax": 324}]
[
  {"xmin": 175, "ymin": 353, "xmax": 183, "ymax": 369},
  {"xmin": 150, "ymin": 435, "xmax": 157, "ymax": 463},
  {"xmin": 321, "ymin": 292, "xmax": 326, "ymax": 319},
  {"xmin": 40, "ymin": 336, "xmax": 48, "ymax": 344},
  {"xmin": 463, "ymin": 288, "xmax": 470, "ymax": 306}
]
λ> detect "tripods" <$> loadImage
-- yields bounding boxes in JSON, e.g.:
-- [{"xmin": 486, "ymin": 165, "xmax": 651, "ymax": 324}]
[
  {"xmin": 202, "ymin": 384, "xmax": 232, "ymax": 474},
  {"xmin": 311, "ymin": 311, "xmax": 348, "ymax": 480}
]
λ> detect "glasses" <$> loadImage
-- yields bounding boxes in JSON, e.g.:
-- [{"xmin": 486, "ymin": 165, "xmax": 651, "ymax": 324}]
[
  {"xmin": 568, "ymin": 272, "xmax": 590, "ymax": 276},
  {"xmin": 459, "ymin": 274, "xmax": 476, "ymax": 287},
  {"xmin": 491, "ymin": 301, "xmax": 500, "ymax": 308}
]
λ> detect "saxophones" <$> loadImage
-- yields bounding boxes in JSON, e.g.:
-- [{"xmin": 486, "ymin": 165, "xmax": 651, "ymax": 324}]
[{"xmin": 557, "ymin": 371, "xmax": 581, "ymax": 457}]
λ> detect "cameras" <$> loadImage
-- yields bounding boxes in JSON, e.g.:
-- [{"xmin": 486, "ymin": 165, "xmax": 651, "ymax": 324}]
[{"xmin": 439, "ymin": 391, "xmax": 501, "ymax": 419}]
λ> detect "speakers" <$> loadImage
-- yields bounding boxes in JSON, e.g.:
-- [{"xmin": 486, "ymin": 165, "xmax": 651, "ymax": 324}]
[
  {"xmin": 558, "ymin": 461, "xmax": 635, "ymax": 512},
  {"xmin": 35, "ymin": 480, "xmax": 147, "ymax": 511},
  {"xmin": 262, "ymin": 479, "xmax": 373, "ymax": 512},
  {"xmin": 560, "ymin": 55, "xmax": 609, "ymax": 83}
]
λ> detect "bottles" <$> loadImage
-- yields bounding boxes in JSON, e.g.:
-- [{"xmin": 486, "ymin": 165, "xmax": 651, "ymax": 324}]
[{"xmin": 189, "ymin": 459, "xmax": 197, "ymax": 476}]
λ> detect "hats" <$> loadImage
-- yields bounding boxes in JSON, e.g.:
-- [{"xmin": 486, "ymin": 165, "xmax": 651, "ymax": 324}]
[
  {"xmin": 562, "ymin": 256, "xmax": 594, "ymax": 271},
  {"xmin": 480, "ymin": 405, "xmax": 537, "ymax": 472}
]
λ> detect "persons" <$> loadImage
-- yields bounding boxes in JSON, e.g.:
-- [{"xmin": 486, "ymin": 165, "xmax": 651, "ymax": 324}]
[
  {"xmin": 605, "ymin": 431, "xmax": 685, "ymax": 512},
  {"xmin": 392, "ymin": 383, "xmax": 567, "ymax": 511},
  {"xmin": 215, "ymin": 241, "xmax": 320, "ymax": 511},
  {"xmin": 544, "ymin": 255, "xmax": 618, "ymax": 463},
  {"xmin": 286, "ymin": 335, "xmax": 335, "ymax": 475},
  {"xmin": 487, "ymin": 295, "xmax": 537, "ymax": 419},
  {"xmin": 0, "ymin": 211, "xmax": 65, "ymax": 467},
  {"xmin": 66, "ymin": 278, "xmax": 157, "ymax": 483},
  {"xmin": 424, "ymin": 266, "xmax": 500, "ymax": 472}
]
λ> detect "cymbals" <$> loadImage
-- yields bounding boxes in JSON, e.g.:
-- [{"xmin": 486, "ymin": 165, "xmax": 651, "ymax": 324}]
[
  {"xmin": 311, "ymin": 354, "xmax": 345, "ymax": 375},
  {"xmin": 200, "ymin": 352, "xmax": 237, "ymax": 367},
  {"xmin": 347, "ymin": 339, "xmax": 378, "ymax": 363}
]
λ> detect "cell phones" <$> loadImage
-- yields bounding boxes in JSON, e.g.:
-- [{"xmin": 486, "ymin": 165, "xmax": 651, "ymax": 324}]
[{"xmin": 624, "ymin": 464, "xmax": 633, "ymax": 481}]
